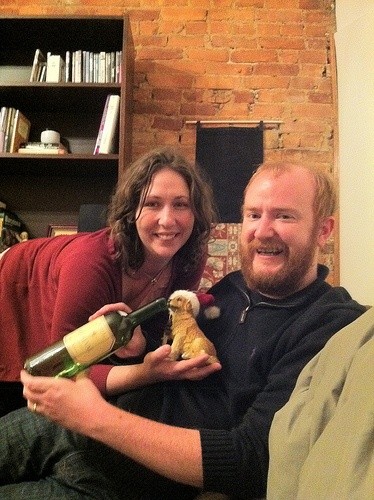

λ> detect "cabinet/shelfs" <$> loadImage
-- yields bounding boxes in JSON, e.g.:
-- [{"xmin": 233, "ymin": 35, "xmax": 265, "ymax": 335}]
[{"xmin": 0, "ymin": 14, "xmax": 134, "ymax": 255}]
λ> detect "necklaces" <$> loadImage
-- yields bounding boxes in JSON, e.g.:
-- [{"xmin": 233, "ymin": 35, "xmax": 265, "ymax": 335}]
[{"xmin": 141, "ymin": 268, "xmax": 163, "ymax": 287}]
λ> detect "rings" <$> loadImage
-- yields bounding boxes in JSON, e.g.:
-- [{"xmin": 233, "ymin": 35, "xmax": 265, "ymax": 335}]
[{"xmin": 33, "ymin": 403, "xmax": 37, "ymax": 413}]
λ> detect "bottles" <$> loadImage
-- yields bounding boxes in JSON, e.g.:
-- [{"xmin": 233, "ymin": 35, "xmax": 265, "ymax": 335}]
[{"xmin": 23, "ymin": 297, "xmax": 168, "ymax": 378}]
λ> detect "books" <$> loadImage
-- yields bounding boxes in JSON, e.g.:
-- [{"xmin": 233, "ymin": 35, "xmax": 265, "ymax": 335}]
[
  {"xmin": 18, "ymin": 142, "xmax": 68, "ymax": 154},
  {"xmin": 30, "ymin": 49, "xmax": 123, "ymax": 84},
  {"xmin": 92, "ymin": 95, "xmax": 120, "ymax": 155},
  {"xmin": 0, "ymin": 107, "xmax": 31, "ymax": 154}
]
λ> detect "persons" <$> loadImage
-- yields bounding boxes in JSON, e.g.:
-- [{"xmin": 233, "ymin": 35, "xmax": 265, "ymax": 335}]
[
  {"xmin": 0, "ymin": 147, "xmax": 222, "ymax": 418},
  {"xmin": 0, "ymin": 159, "xmax": 368, "ymax": 500}
]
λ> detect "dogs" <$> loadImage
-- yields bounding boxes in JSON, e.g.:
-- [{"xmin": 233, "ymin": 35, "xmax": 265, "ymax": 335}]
[{"xmin": 165, "ymin": 289, "xmax": 220, "ymax": 366}]
